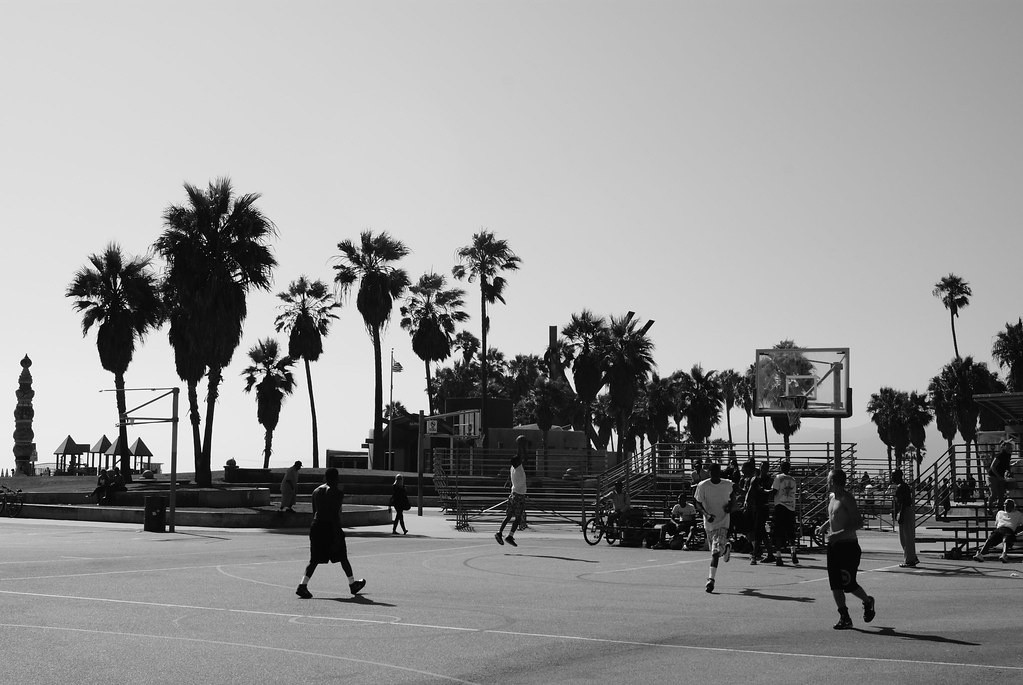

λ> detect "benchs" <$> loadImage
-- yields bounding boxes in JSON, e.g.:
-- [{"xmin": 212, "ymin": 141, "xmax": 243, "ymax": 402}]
[
  {"xmin": 621, "ymin": 482, "xmax": 828, "ymax": 524},
  {"xmin": 433, "ymin": 447, "xmax": 608, "ymax": 523},
  {"xmin": 616, "ymin": 526, "xmax": 801, "ymax": 546},
  {"xmin": 919, "ymin": 484, "xmax": 1023, "ymax": 558}
]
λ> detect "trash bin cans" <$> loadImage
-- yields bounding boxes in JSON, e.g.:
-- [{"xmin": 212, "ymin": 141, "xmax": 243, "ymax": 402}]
[{"xmin": 145, "ymin": 496, "xmax": 167, "ymax": 532}]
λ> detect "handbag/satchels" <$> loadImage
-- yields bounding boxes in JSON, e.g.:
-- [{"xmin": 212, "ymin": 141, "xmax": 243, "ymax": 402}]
[{"xmin": 403, "ymin": 497, "xmax": 411, "ymax": 510}]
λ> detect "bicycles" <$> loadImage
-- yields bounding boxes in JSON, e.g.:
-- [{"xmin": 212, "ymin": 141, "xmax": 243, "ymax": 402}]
[
  {"xmin": 583, "ymin": 502, "xmax": 618, "ymax": 545},
  {"xmin": 799, "ymin": 516, "xmax": 830, "ymax": 548}
]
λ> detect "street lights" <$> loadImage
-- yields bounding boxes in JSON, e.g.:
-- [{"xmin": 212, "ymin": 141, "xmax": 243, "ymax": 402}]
[{"xmin": 388, "ymin": 361, "xmax": 403, "ymax": 471}]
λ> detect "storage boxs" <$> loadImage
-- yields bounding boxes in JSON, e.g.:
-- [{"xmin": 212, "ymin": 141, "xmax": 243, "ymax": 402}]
[{"xmin": 144, "ymin": 496, "xmax": 166, "ymax": 532}]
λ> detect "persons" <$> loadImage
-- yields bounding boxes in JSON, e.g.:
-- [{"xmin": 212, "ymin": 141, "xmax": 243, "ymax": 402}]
[
  {"xmin": 650, "ymin": 459, "xmax": 800, "ymax": 565},
  {"xmin": 602, "ymin": 482, "xmax": 631, "ymax": 538},
  {"xmin": 295, "ymin": 467, "xmax": 366, "ymax": 599},
  {"xmin": 972, "ymin": 499, "xmax": 1023, "ymax": 564},
  {"xmin": 815, "ymin": 469, "xmax": 875, "ymax": 630},
  {"xmin": 694, "ymin": 462, "xmax": 738, "ymax": 592},
  {"xmin": 986, "ymin": 441, "xmax": 1014, "ymax": 514},
  {"xmin": 84, "ymin": 467, "xmax": 125, "ymax": 505},
  {"xmin": 389, "ymin": 473, "xmax": 409, "ymax": 535},
  {"xmin": 279, "ymin": 461, "xmax": 302, "ymax": 513},
  {"xmin": 494, "ymin": 439, "xmax": 528, "ymax": 547},
  {"xmin": 927, "ymin": 474, "xmax": 977, "ymax": 520},
  {"xmin": 891, "ymin": 470, "xmax": 920, "ymax": 568},
  {"xmin": 859, "ymin": 472, "xmax": 871, "ymax": 493}
]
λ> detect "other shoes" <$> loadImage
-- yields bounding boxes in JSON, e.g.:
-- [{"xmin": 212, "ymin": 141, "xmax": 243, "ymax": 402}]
[
  {"xmin": 404, "ymin": 530, "xmax": 408, "ymax": 535},
  {"xmin": 833, "ymin": 617, "xmax": 853, "ymax": 629},
  {"xmin": 724, "ymin": 542, "xmax": 732, "ymax": 562},
  {"xmin": 505, "ymin": 535, "xmax": 517, "ymax": 547},
  {"xmin": 393, "ymin": 531, "xmax": 399, "ymax": 534},
  {"xmin": 973, "ymin": 551, "xmax": 984, "ymax": 562},
  {"xmin": 776, "ymin": 560, "xmax": 783, "ymax": 566},
  {"xmin": 749, "ymin": 557, "xmax": 756, "ymax": 565},
  {"xmin": 296, "ymin": 583, "xmax": 313, "ymax": 599},
  {"xmin": 706, "ymin": 578, "xmax": 715, "ymax": 593},
  {"xmin": 862, "ymin": 596, "xmax": 875, "ymax": 622},
  {"xmin": 495, "ymin": 532, "xmax": 504, "ymax": 545},
  {"xmin": 792, "ymin": 557, "xmax": 798, "ymax": 564},
  {"xmin": 899, "ymin": 556, "xmax": 919, "ymax": 567},
  {"xmin": 350, "ymin": 579, "xmax": 366, "ymax": 594},
  {"xmin": 1000, "ymin": 555, "xmax": 1008, "ymax": 563},
  {"xmin": 761, "ymin": 556, "xmax": 775, "ymax": 562}
]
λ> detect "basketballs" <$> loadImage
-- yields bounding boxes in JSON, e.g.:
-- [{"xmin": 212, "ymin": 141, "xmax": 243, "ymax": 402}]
[
  {"xmin": 516, "ymin": 434, "xmax": 528, "ymax": 447},
  {"xmin": 670, "ymin": 539, "xmax": 683, "ymax": 550}
]
